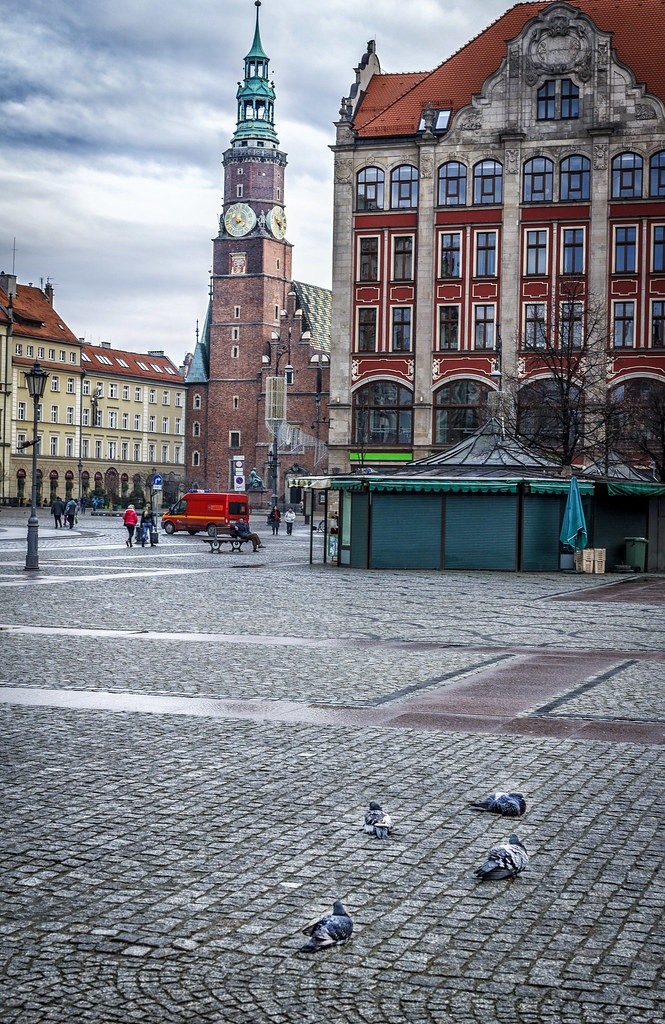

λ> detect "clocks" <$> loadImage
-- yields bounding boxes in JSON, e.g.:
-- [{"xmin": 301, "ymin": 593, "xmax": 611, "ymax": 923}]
[
  {"xmin": 225, "ymin": 203, "xmax": 256, "ymax": 236},
  {"xmin": 266, "ymin": 205, "xmax": 288, "ymax": 240}
]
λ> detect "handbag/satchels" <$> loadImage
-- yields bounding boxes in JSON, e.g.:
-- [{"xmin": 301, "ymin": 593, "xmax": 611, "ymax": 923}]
[
  {"xmin": 150, "ymin": 526, "xmax": 158, "ymax": 543},
  {"xmin": 64, "ymin": 514, "xmax": 67, "ymax": 523},
  {"xmin": 75, "ymin": 513, "xmax": 78, "ymax": 524}
]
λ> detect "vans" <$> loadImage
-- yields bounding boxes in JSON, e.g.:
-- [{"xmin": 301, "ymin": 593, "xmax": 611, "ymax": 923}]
[{"xmin": 161, "ymin": 492, "xmax": 252, "ymax": 536}]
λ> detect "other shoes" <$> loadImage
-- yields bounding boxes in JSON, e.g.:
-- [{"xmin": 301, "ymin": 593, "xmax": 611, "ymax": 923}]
[
  {"xmin": 253, "ymin": 550, "xmax": 259, "ymax": 552},
  {"xmin": 151, "ymin": 545, "xmax": 156, "ymax": 547},
  {"xmin": 129, "ymin": 544, "xmax": 133, "ymax": 547},
  {"xmin": 258, "ymin": 545, "xmax": 266, "ymax": 548},
  {"xmin": 126, "ymin": 540, "xmax": 129, "ymax": 546},
  {"xmin": 142, "ymin": 543, "xmax": 145, "ymax": 547}
]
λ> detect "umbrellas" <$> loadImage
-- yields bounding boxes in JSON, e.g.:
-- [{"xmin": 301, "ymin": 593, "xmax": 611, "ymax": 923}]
[{"xmin": 559, "ymin": 476, "xmax": 588, "ymax": 570}]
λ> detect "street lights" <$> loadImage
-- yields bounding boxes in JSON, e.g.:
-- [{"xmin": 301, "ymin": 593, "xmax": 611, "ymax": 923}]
[
  {"xmin": 24, "ymin": 358, "xmax": 49, "ymax": 571},
  {"xmin": 265, "ymin": 344, "xmax": 293, "ymax": 519}
]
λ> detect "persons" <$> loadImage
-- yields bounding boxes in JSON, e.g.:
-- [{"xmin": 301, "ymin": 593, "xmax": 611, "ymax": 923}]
[
  {"xmin": 50, "ymin": 496, "xmax": 64, "ymax": 529},
  {"xmin": 140, "ymin": 503, "xmax": 157, "ymax": 547},
  {"xmin": 248, "ymin": 467, "xmax": 267, "ymax": 489},
  {"xmin": 81, "ymin": 495, "xmax": 88, "ymax": 514},
  {"xmin": 270, "ymin": 506, "xmax": 281, "ymax": 536},
  {"xmin": 91, "ymin": 495, "xmax": 104, "ymax": 511},
  {"xmin": 63, "ymin": 496, "xmax": 80, "ymax": 529},
  {"xmin": 284, "ymin": 507, "xmax": 295, "ymax": 536},
  {"xmin": 122, "ymin": 504, "xmax": 138, "ymax": 548},
  {"xmin": 300, "ymin": 498, "xmax": 305, "ymax": 513},
  {"xmin": 230, "ymin": 517, "xmax": 266, "ymax": 552}
]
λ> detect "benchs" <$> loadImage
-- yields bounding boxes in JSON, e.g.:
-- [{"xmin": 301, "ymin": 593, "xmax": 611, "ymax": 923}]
[{"xmin": 203, "ymin": 528, "xmax": 248, "ymax": 553}]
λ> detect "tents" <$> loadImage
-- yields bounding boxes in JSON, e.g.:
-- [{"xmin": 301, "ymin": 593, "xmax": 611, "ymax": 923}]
[
  {"xmin": 288, "ymin": 414, "xmax": 595, "ymax": 573},
  {"xmin": 575, "ymin": 449, "xmax": 665, "ymax": 573}
]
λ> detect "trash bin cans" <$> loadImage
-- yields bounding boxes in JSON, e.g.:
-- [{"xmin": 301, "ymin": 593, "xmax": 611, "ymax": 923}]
[{"xmin": 624, "ymin": 537, "xmax": 650, "ymax": 572}]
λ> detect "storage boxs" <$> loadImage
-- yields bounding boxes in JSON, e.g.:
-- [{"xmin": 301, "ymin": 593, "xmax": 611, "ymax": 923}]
[{"xmin": 574, "ymin": 548, "xmax": 606, "ymax": 573}]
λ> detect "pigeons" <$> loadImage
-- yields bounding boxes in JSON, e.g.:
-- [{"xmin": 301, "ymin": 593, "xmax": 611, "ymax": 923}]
[
  {"xmin": 472, "ymin": 834, "xmax": 529, "ymax": 881},
  {"xmin": 364, "ymin": 802, "xmax": 392, "ymax": 839},
  {"xmin": 469, "ymin": 792, "xmax": 526, "ymax": 816},
  {"xmin": 302, "ymin": 901, "xmax": 353, "ymax": 945}
]
